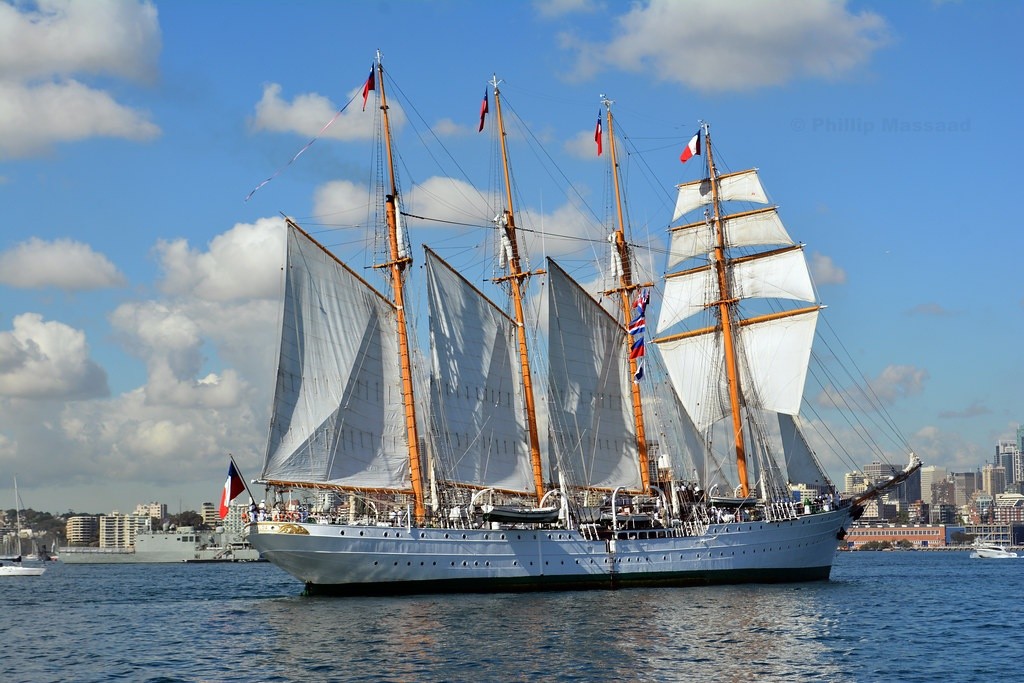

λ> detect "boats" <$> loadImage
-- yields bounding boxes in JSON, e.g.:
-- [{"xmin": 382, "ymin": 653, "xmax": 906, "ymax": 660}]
[
  {"xmin": 58, "ymin": 525, "xmax": 268, "ymax": 563},
  {"xmin": 481, "ymin": 503, "xmax": 560, "ymax": 517},
  {"xmin": 710, "ymin": 497, "xmax": 757, "ymax": 503},
  {"xmin": 977, "ymin": 546, "xmax": 1017, "ymax": 558}
]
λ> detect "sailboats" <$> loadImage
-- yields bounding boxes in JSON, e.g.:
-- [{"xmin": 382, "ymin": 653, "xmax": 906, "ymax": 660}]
[
  {"xmin": 242, "ymin": 49, "xmax": 921, "ymax": 598},
  {"xmin": 0, "ymin": 472, "xmax": 49, "ymax": 575}
]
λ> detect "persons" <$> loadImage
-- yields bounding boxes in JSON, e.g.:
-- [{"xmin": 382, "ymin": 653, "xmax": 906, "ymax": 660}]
[
  {"xmin": 259, "ymin": 499, "xmax": 267, "ymax": 515},
  {"xmin": 822, "ymin": 491, "xmax": 840, "ymax": 510},
  {"xmin": 391, "ymin": 507, "xmax": 404, "ymax": 527}
]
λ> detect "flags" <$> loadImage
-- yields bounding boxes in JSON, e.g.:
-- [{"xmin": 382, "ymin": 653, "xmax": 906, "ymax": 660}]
[
  {"xmin": 219, "ymin": 461, "xmax": 245, "ymax": 519},
  {"xmin": 628, "ymin": 290, "xmax": 650, "ymax": 385},
  {"xmin": 363, "ymin": 62, "xmax": 375, "ymax": 112},
  {"xmin": 680, "ymin": 130, "xmax": 701, "ymax": 162},
  {"xmin": 478, "ymin": 85, "xmax": 488, "ymax": 132},
  {"xmin": 594, "ymin": 108, "xmax": 602, "ymax": 156}
]
